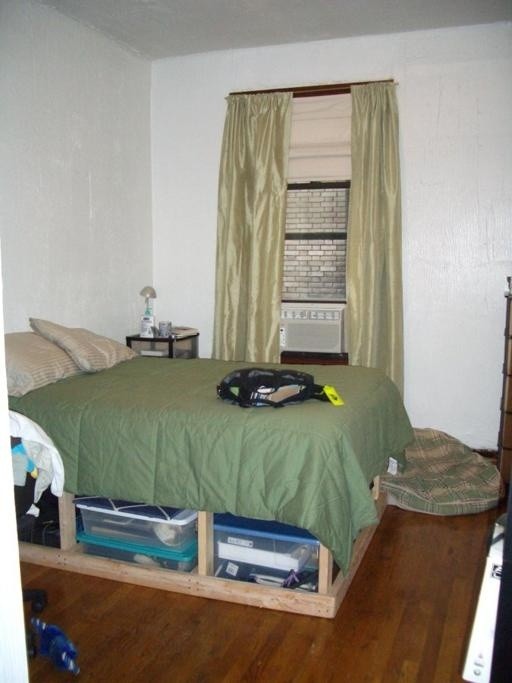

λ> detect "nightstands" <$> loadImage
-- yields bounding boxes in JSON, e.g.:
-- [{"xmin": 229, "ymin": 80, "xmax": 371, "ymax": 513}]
[{"xmin": 126, "ymin": 329, "xmax": 200, "ymax": 358}]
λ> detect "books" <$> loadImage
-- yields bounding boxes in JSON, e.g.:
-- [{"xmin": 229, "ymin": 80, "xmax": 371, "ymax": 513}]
[
  {"xmin": 141, "ymin": 347, "xmax": 176, "ymax": 357},
  {"xmin": 170, "ymin": 325, "xmax": 198, "ymax": 339},
  {"xmin": 215, "ymin": 531, "xmax": 320, "ymax": 593}
]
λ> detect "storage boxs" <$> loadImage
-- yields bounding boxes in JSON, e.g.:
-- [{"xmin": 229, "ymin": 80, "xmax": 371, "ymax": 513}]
[
  {"xmin": 212, "ymin": 514, "xmax": 320, "ymax": 584},
  {"xmin": 73, "ymin": 497, "xmax": 196, "ymax": 551},
  {"xmin": 76, "ymin": 531, "xmax": 196, "ymax": 572}
]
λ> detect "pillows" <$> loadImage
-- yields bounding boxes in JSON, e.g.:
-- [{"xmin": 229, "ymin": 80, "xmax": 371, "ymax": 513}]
[{"xmin": 4, "ymin": 319, "xmax": 139, "ymax": 397}]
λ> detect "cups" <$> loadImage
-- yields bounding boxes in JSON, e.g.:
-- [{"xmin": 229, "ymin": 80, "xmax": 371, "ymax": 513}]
[{"xmin": 159, "ymin": 320, "xmax": 171, "ymax": 336}]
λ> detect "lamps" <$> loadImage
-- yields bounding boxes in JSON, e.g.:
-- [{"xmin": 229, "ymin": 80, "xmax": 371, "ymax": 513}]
[{"xmin": 140, "ymin": 285, "xmax": 157, "ymax": 317}]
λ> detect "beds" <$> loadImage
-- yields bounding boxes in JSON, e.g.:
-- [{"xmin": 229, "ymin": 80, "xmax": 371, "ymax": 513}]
[{"xmin": 8, "ymin": 356, "xmax": 417, "ymax": 618}]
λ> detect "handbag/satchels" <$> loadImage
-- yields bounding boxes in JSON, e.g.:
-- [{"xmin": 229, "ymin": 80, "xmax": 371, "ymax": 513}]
[{"xmin": 216, "ymin": 367, "xmax": 331, "ymax": 407}]
